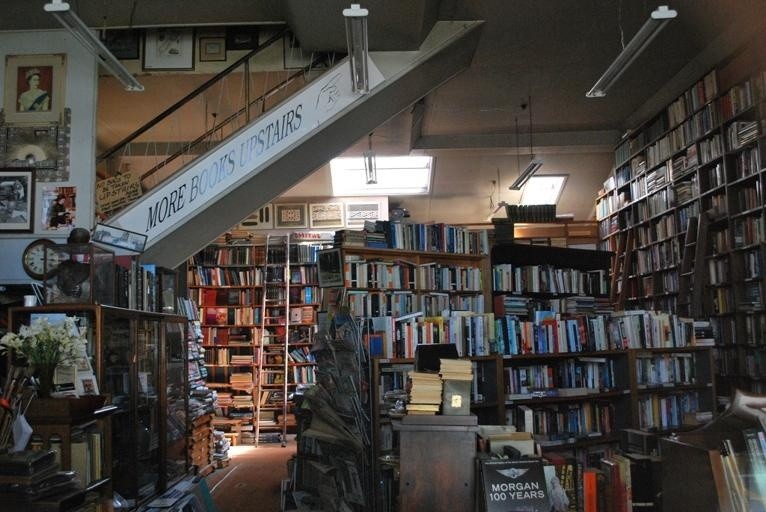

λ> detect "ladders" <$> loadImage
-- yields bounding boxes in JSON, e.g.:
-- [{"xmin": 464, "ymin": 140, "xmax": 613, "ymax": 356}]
[
  {"xmin": 610, "ymin": 229, "xmax": 635, "ymax": 311},
  {"xmin": 254, "ymin": 232, "xmax": 290, "ymax": 449},
  {"xmin": 676, "ymin": 211, "xmax": 709, "ymax": 320}
]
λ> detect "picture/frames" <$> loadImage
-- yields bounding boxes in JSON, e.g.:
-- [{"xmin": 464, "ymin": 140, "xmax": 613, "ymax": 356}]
[{"xmin": 0, "ymin": 167, "xmax": 36, "ymax": 233}]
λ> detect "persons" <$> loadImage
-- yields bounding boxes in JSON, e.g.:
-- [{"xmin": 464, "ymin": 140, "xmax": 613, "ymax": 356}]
[{"xmin": 48, "ymin": 194, "xmax": 69, "ymax": 228}]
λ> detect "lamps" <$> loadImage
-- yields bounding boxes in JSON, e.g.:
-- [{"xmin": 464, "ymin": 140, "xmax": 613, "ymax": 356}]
[
  {"xmin": 43, "ymin": 0, "xmax": 146, "ymax": 92},
  {"xmin": 585, "ymin": 0, "xmax": 678, "ymax": 99},
  {"xmin": 342, "ymin": 0, "xmax": 371, "ymax": 96}
]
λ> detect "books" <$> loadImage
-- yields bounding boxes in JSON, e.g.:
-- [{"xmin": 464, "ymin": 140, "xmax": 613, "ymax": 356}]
[
  {"xmin": 283, "ymin": 218, "xmax": 608, "ymax": 511},
  {"xmin": 1, "ymin": 254, "xmax": 233, "ymax": 512},
  {"xmin": 188, "ymin": 244, "xmax": 322, "ymax": 444},
  {"xmin": 593, "ymin": 71, "xmax": 766, "ymax": 512}
]
